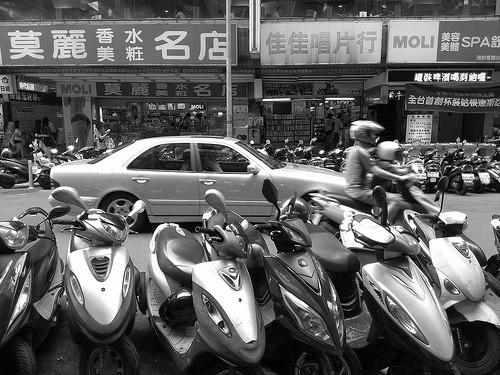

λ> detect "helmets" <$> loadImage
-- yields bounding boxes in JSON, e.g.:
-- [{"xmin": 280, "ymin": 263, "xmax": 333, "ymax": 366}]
[
  {"xmin": 375, "ymin": 141, "xmax": 402, "ymax": 161},
  {"xmin": 275, "ymin": 197, "xmax": 312, "ymax": 223},
  {"xmin": 159, "ymin": 287, "xmax": 215, "ymax": 325},
  {"xmin": 350, "ymin": 121, "xmax": 385, "ymax": 145},
  {"xmin": 484, "ymin": 254, "xmax": 500, "ymax": 279}
]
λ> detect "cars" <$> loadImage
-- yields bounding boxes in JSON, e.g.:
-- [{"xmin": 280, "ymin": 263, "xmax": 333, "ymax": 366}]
[{"xmin": 50, "ymin": 135, "xmax": 348, "ymax": 234}]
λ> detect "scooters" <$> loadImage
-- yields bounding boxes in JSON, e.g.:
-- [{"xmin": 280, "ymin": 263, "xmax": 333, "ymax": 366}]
[
  {"xmin": 394, "ymin": 208, "xmax": 500, "ymax": 317},
  {"xmin": 0, "ymin": 134, "xmax": 55, "ymax": 190},
  {"xmin": 30, "ymin": 125, "xmax": 115, "ymax": 189},
  {"xmin": 0, "ymin": 208, "xmax": 65, "ymax": 375},
  {"xmin": 308, "ymin": 141, "xmax": 441, "ymax": 241},
  {"xmin": 51, "ymin": 187, "xmax": 148, "ymax": 375},
  {"xmin": 202, "ymin": 179, "xmax": 351, "ymax": 375},
  {"xmin": 393, "ymin": 175, "xmax": 500, "ymax": 375},
  {"xmin": 146, "ymin": 188, "xmax": 270, "ymax": 375},
  {"xmin": 248, "ymin": 136, "xmax": 500, "ymax": 195},
  {"xmin": 301, "ymin": 185, "xmax": 456, "ymax": 375}
]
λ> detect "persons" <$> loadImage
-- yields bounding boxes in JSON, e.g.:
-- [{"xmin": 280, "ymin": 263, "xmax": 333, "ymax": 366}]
[
  {"xmin": 270, "ymin": 4, "xmax": 285, "ymax": 18},
  {"xmin": 345, "ymin": 120, "xmax": 420, "ymax": 208},
  {"xmin": 180, "ymin": 146, "xmax": 224, "ymax": 173},
  {"xmin": 181, "ymin": 111, "xmax": 205, "ymax": 134},
  {"xmin": 323, "ymin": 112, "xmax": 344, "ymax": 152},
  {"xmin": 173, "ymin": 5, "xmax": 188, "ymax": 18},
  {"xmin": 9, "ymin": 116, "xmax": 58, "ymax": 160}
]
